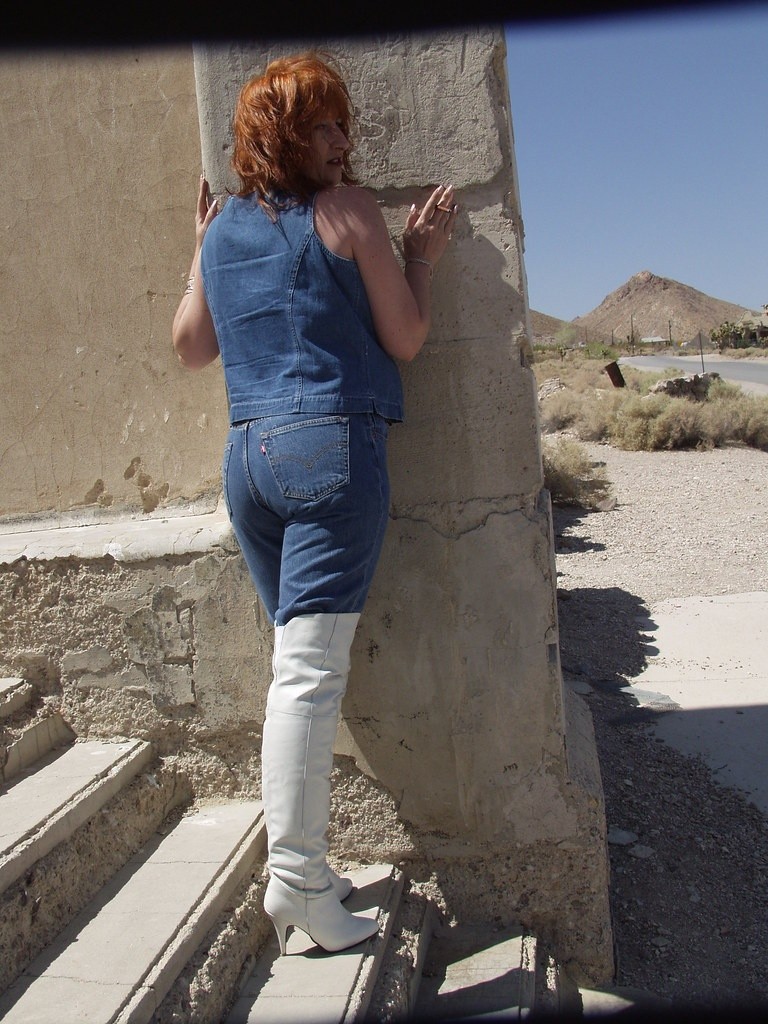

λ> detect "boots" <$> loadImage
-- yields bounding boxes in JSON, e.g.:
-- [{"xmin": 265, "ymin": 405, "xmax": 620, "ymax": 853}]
[
  {"xmin": 321, "ymin": 858, "xmax": 352, "ymax": 903},
  {"xmin": 263, "ymin": 612, "xmax": 380, "ymax": 957}
]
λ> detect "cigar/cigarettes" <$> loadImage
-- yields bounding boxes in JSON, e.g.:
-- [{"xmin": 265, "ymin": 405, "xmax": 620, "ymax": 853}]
[{"xmin": 436, "ymin": 206, "xmax": 452, "ymax": 213}]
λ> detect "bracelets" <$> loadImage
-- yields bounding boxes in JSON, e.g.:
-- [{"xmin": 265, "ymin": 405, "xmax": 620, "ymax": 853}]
[
  {"xmin": 404, "ymin": 259, "xmax": 434, "ymax": 280},
  {"xmin": 185, "ymin": 278, "xmax": 195, "ymax": 294}
]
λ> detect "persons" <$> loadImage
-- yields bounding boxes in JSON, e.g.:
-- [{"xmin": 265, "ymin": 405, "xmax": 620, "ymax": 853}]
[{"xmin": 171, "ymin": 57, "xmax": 459, "ymax": 956}]
